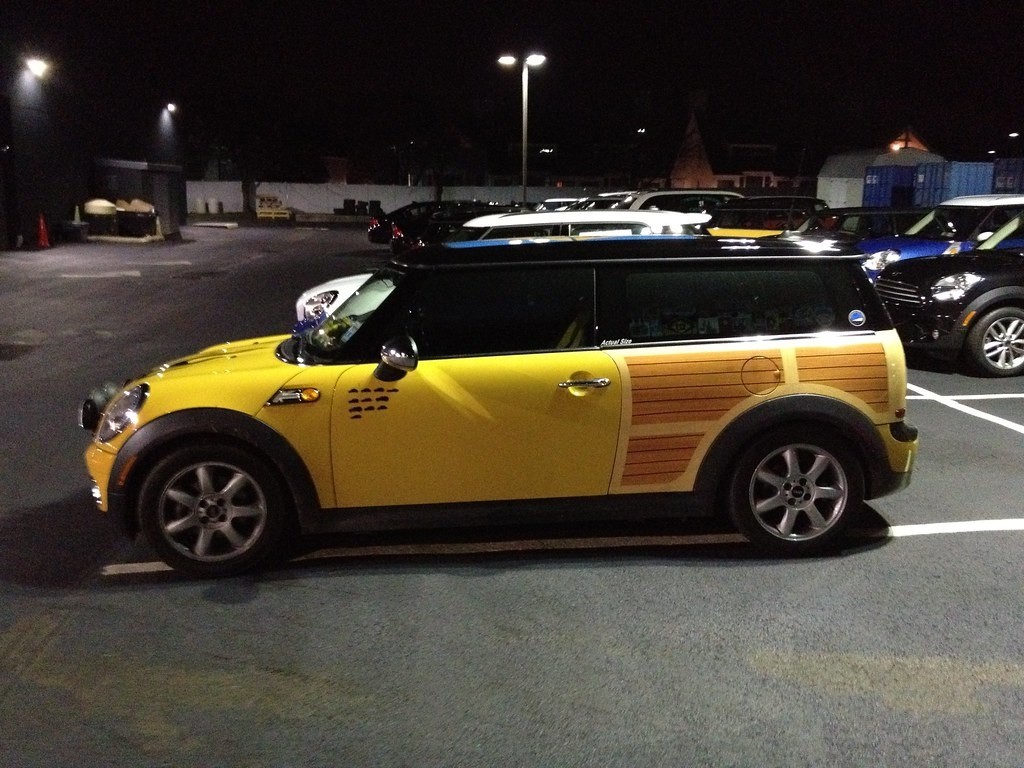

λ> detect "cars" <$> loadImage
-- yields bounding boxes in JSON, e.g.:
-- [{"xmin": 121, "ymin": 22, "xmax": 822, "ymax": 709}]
[
  {"xmin": 75, "ymin": 228, "xmax": 921, "ymax": 582},
  {"xmin": 364, "ymin": 184, "xmax": 1024, "ymax": 380}
]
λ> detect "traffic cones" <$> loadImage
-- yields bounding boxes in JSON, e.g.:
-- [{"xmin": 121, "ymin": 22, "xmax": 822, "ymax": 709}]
[
  {"xmin": 73, "ymin": 206, "xmax": 82, "ymax": 225},
  {"xmin": 35, "ymin": 214, "xmax": 51, "ymax": 248}
]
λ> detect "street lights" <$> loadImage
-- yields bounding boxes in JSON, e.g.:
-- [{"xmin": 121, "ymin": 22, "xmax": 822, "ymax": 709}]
[{"xmin": 496, "ymin": 51, "xmax": 547, "ymax": 209}]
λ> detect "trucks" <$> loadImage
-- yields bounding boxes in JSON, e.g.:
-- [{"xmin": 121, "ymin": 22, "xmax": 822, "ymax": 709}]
[{"xmin": 257, "ymin": 194, "xmax": 291, "ymax": 225}]
